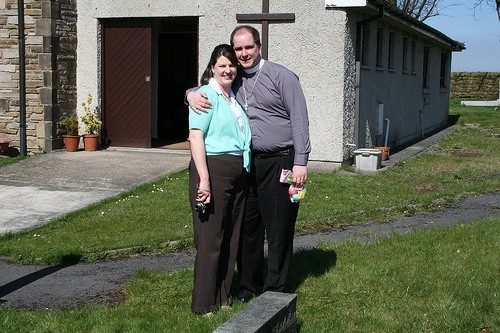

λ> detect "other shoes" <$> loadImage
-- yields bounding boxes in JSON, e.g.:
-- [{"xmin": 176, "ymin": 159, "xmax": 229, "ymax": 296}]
[
  {"xmin": 220, "ymin": 305, "xmax": 232, "ymax": 312},
  {"xmin": 194, "ymin": 312, "xmax": 215, "ymax": 319}
]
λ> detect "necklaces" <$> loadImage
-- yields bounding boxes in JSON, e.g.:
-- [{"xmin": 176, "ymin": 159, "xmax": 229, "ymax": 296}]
[
  {"xmin": 241, "ymin": 59, "xmax": 263, "ymax": 110},
  {"xmin": 222, "ymin": 92, "xmax": 231, "ymax": 101}
]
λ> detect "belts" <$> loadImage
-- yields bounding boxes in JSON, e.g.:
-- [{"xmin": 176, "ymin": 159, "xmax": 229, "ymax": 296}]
[{"xmin": 251, "ymin": 146, "xmax": 294, "ymax": 157}]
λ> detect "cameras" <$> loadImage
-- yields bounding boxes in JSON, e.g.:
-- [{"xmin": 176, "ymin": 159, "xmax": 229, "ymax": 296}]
[{"xmin": 194, "ymin": 201, "xmax": 206, "ymax": 214}]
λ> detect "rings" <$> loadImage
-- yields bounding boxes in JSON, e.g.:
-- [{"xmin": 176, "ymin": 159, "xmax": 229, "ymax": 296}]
[{"xmin": 300, "ymin": 179, "xmax": 304, "ymax": 180}]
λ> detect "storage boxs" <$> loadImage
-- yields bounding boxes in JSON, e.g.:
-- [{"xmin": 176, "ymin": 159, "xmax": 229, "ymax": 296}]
[
  {"xmin": 375, "ymin": 147, "xmax": 390, "ymax": 160},
  {"xmin": 354, "ymin": 148, "xmax": 383, "ymax": 170}
]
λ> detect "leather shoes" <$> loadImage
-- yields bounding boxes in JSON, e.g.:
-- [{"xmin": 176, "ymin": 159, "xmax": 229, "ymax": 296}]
[{"xmin": 237, "ymin": 296, "xmax": 253, "ymax": 302}]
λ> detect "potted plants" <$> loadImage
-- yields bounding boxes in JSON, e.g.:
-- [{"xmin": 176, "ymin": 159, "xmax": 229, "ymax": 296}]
[
  {"xmin": 80, "ymin": 93, "xmax": 102, "ymax": 151},
  {"xmin": 59, "ymin": 114, "xmax": 81, "ymax": 152}
]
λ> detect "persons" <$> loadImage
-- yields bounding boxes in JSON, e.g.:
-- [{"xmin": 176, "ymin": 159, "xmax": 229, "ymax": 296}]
[
  {"xmin": 188, "ymin": 44, "xmax": 251, "ymax": 319},
  {"xmin": 183, "ymin": 25, "xmax": 310, "ymax": 304}
]
